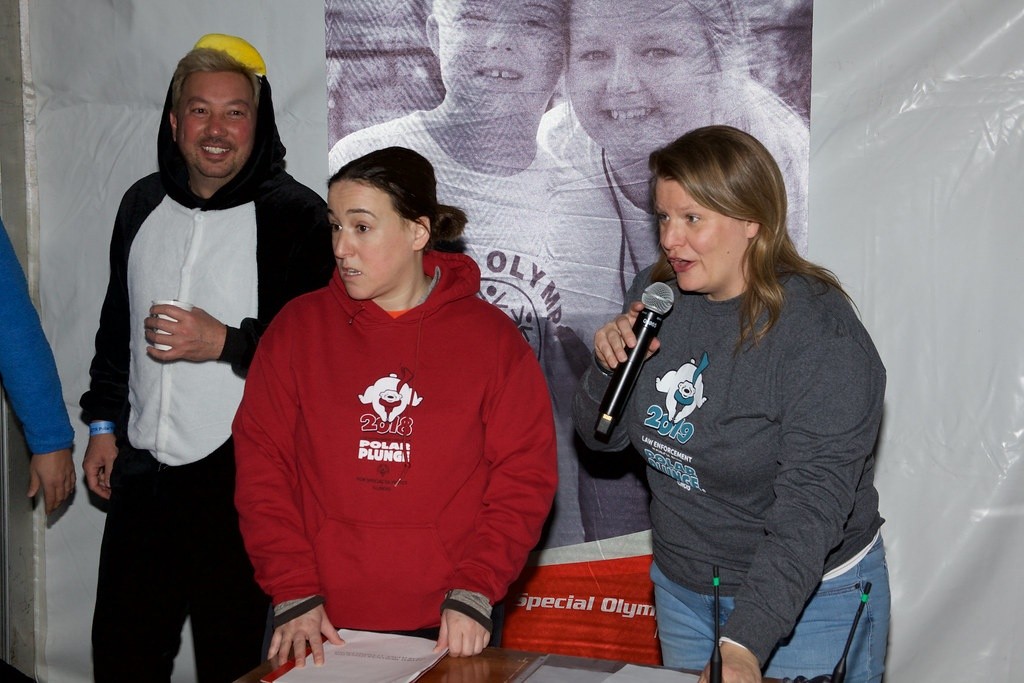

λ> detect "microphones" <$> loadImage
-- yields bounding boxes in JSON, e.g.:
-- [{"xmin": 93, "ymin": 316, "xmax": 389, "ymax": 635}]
[{"xmin": 596, "ymin": 281, "xmax": 674, "ymax": 435}]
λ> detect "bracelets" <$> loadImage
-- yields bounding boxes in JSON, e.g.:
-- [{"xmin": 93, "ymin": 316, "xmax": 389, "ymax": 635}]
[{"xmin": 89, "ymin": 421, "xmax": 116, "ymax": 437}]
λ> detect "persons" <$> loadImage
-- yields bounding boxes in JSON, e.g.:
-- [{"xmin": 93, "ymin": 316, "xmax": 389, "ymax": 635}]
[
  {"xmin": 535, "ymin": 0, "xmax": 812, "ymax": 308},
  {"xmin": 226, "ymin": 144, "xmax": 561, "ymax": 674},
  {"xmin": 0, "ymin": 217, "xmax": 80, "ymax": 517},
  {"xmin": 327, "ymin": 1, "xmax": 641, "ymax": 556},
  {"xmin": 571, "ymin": 122, "xmax": 894, "ymax": 681},
  {"xmin": 65, "ymin": 45, "xmax": 335, "ymax": 681}
]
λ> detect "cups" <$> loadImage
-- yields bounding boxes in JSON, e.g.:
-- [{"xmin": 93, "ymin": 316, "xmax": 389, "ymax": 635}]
[{"xmin": 151, "ymin": 299, "xmax": 193, "ymax": 351}]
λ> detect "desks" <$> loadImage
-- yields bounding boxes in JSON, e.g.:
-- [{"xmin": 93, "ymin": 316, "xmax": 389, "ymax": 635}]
[{"xmin": 231, "ymin": 628, "xmax": 796, "ymax": 683}]
